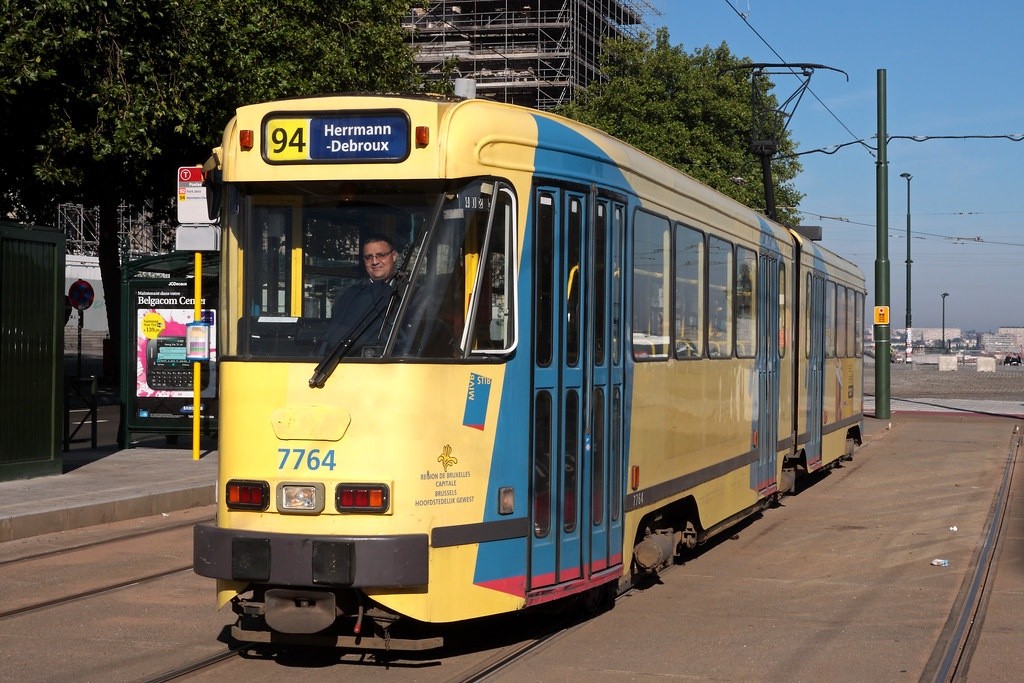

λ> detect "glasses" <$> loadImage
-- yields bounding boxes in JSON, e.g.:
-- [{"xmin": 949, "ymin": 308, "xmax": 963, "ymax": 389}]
[{"xmin": 362, "ymin": 251, "xmax": 393, "ymax": 261}]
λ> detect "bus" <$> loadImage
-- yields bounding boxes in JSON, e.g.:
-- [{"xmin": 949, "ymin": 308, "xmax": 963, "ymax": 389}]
[{"xmin": 193, "ymin": 96, "xmax": 867, "ymax": 654}]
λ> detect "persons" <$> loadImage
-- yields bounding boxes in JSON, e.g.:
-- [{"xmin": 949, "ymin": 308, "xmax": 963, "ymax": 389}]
[
  {"xmin": 331, "ymin": 233, "xmax": 425, "ymax": 341},
  {"xmin": 1004, "ymin": 353, "xmax": 1021, "ymax": 366},
  {"xmin": 890, "ymin": 344, "xmax": 893, "ymax": 360}
]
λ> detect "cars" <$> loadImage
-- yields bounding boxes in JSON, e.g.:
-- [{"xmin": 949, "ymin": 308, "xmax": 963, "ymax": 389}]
[{"xmin": 64, "ymin": 353, "xmax": 120, "ymax": 409}]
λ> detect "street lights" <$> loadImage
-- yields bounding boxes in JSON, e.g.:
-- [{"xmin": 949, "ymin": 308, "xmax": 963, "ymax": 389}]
[
  {"xmin": 941, "ymin": 293, "xmax": 949, "ymax": 346},
  {"xmin": 900, "ymin": 173, "xmax": 913, "ymax": 364}
]
